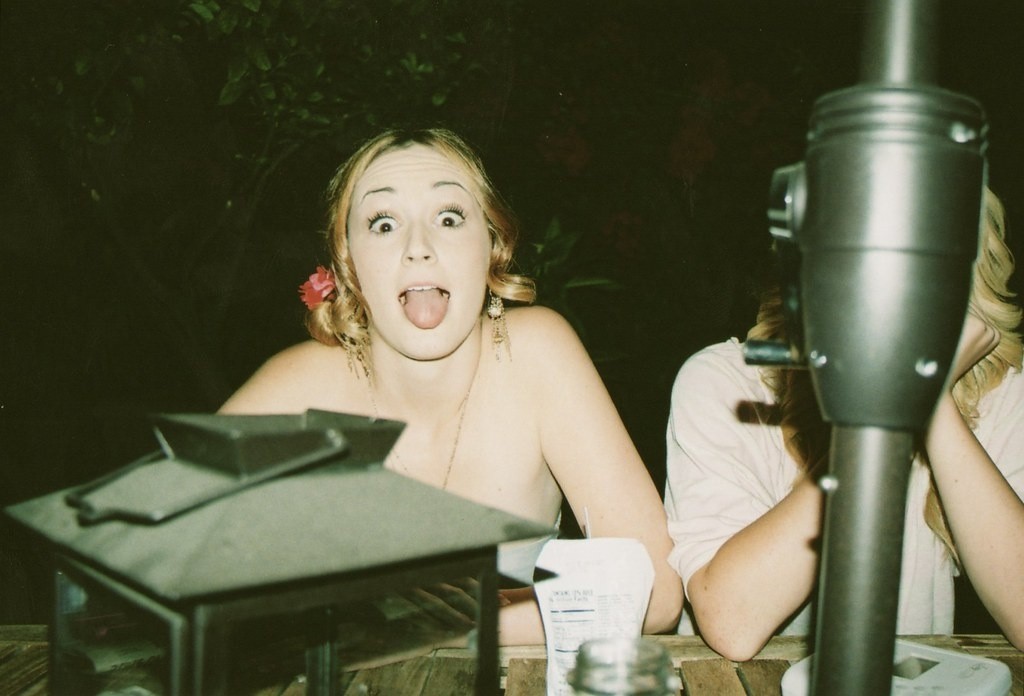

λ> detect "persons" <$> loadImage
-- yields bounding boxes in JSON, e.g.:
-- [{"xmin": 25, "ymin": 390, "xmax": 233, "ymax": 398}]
[
  {"xmin": 210, "ymin": 124, "xmax": 683, "ymax": 676},
  {"xmin": 665, "ymin": 187, "xmax": 1022, "ymax": 662}
]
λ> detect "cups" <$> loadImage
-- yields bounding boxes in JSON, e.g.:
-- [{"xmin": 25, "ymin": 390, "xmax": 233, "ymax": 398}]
[{"xmin": 566, "ymin": 637, "xmax": 680, "ymax": 696}]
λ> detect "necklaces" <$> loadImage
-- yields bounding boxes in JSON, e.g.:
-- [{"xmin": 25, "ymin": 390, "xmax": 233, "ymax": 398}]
[{"xmin": 366, "ymin": 315, "xmax": 484, "ymax": 489}]
